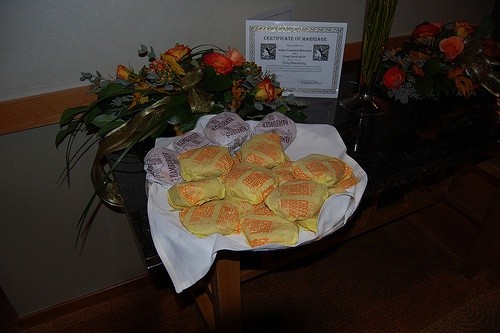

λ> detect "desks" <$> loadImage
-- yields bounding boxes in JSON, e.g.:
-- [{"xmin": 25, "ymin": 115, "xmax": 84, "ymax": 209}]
[{"xmin": 100, "ymin": 50, "xmax": 500, "ymax": 333}]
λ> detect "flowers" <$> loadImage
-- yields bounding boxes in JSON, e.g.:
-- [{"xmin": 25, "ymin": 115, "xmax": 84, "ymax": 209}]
[
  {"xmin": 374, "ymin": 16, "xmax": 500, "ymax": 139},
  {"xmin": 52, "ymin": 41, "xmax": 312, "ymax": 247}
]
